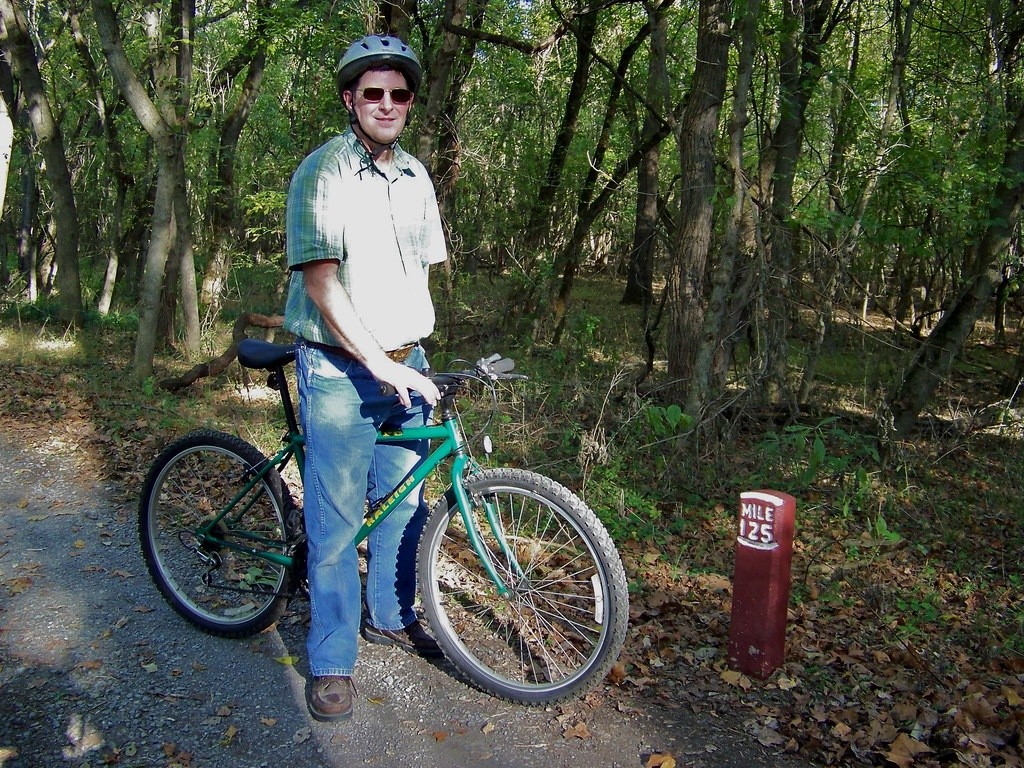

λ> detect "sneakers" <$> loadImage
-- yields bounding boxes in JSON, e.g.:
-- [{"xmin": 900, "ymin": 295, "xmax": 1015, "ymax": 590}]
[
  {"xmin": 308, "ymin": 675, "xmax": 358, "ymax": 721},
  {"xmin": 365, "ymin": 615, "xmax": 444, "ymax": 658}
]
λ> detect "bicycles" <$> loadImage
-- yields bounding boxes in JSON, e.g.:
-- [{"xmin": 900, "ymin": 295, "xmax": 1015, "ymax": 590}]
[{"xmin": 138, "ymin": 339, "xmax": 630, "ymax": 705}]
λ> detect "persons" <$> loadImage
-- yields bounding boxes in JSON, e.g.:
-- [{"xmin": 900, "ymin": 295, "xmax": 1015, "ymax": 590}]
[{"xmin": 282, "ymin": 34, "xmax": 447, "ymax": 723}]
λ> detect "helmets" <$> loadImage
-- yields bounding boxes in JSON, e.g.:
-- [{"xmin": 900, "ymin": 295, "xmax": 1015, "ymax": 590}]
[{"xmin": 337, "ymin": 36, "xmax": 423, "ymax": 91}]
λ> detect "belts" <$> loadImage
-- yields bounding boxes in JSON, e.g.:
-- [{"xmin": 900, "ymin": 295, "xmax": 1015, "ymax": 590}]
[{"xmin": 301, "ymin": 337, "xmax": 418, "ymax": 363}]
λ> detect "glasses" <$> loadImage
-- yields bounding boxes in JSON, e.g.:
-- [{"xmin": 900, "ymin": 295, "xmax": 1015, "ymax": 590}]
[{"xmin": 347, "ymin": 87, "xmax": 413, "ymax": 104}]
